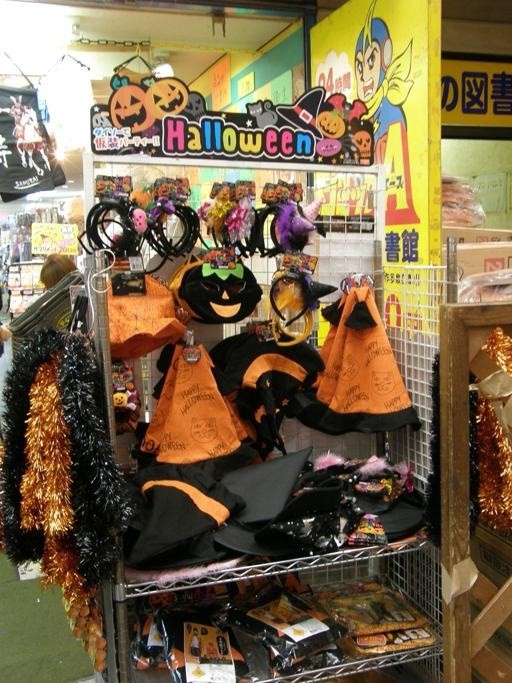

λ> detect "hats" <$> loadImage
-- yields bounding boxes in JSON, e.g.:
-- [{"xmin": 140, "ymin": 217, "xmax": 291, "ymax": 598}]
[
  {"xmin": 275, "ymin": 83, "xmax": 326, "ymax": 141},
  {"xmin": 132, "ymin": 338, "xmax": 258, "ymax": 479},
  {"xmin": 297, "ymin": 286, "xmax": 420, "ymax": 436},
  {"xmin": 126, "ymin": 463, "xmax": 247, "ymax": 566},
  {"xmin": 209, "ymin": 331, "xmax": 325, "ymax": 462}
]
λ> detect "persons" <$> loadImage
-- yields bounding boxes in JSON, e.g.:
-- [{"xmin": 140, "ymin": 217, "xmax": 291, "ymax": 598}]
[
  {"xmin": 351, "ymin": 0, "xmax": 423, "ymax": 224},
  {"xmin": 0, "ymin": 89, "xmax": 53, "ymax": 176}
]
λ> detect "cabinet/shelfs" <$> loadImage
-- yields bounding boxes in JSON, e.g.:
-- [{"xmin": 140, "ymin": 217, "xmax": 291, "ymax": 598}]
[{"xmin": 84, "ymin": 155, "xmax": 444, "ymax": 678}]
